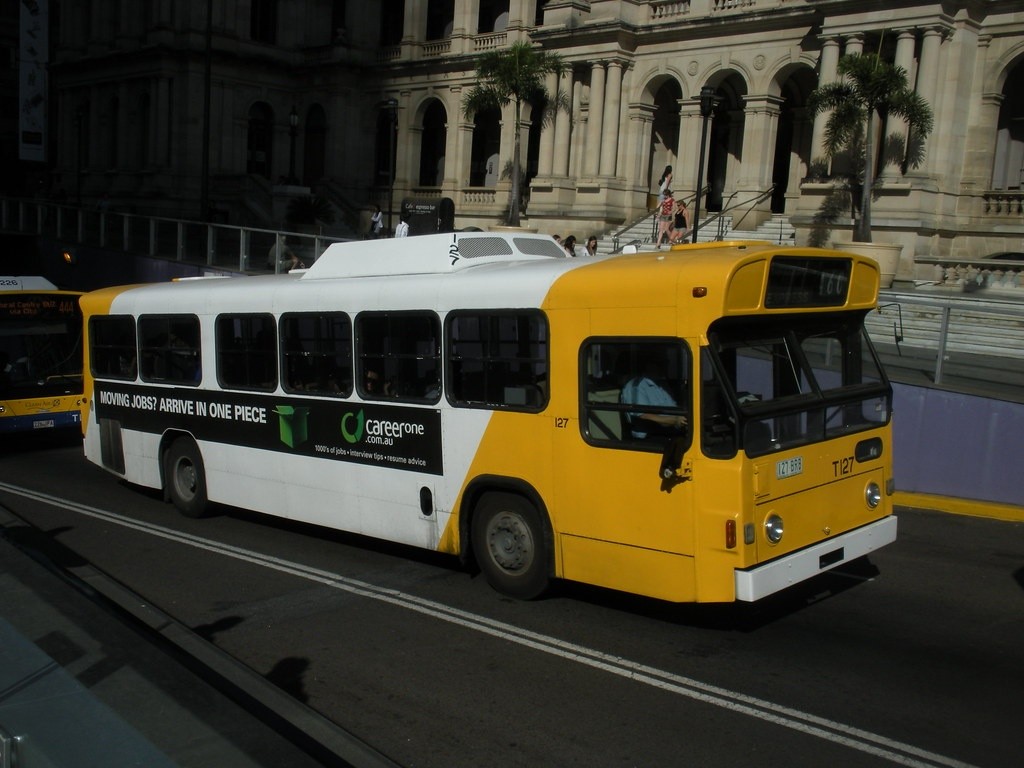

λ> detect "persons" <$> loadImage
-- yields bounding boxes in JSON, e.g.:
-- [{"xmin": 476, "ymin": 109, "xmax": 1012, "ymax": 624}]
[
  {"xmin": 657, "ymin": 165, "xmax": 673, "ymax": 203},
  {"xmin": 228, "ymin": 327, "xmax": 546, "ymax": 407},
  {"xmin": 394, "ymin": 214, "xmax": 409, "ymax": 238},
  {"xmin": 269, "ymin": 235, "xmax": 302, "ymax": 274},
  {"xmin": 655, "ymin": 189, "xmax": 674, "ymax": 247},
  {"xmin": 670, "ymin": 201, "xmax": 690, "ymax": 243},
  {"xmin": 552, "ymin": 235, "xmax": 560, "ymax": 243},
  {"xmin": 622, "ymin": 346, "xmax": 688, "ymax": 434},
  {"xmin": 368, "ymin": 205, "xmax": 383, "ymax": 237},
  {"xmin": 585, "ymin": 236, "xmax": 597, "ymax": 255},
  {"xmin": 564, "ymin": 235, "xmax": 576, "ymax": 257}
]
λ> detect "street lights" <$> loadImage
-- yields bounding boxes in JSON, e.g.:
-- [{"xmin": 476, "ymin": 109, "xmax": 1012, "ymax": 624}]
[{"xmin": 691, "ymin": 85, "xmax": 724, "ymax": 244}]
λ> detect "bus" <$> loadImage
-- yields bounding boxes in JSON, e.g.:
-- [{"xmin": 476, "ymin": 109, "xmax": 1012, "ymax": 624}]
[
  {"xmin": 77, "ymin": 223, "xmax": 905, "ymax": 609},
  {"xmin": 0, "ymin": 275, "xmax": 100, "ymax": 437}
]
